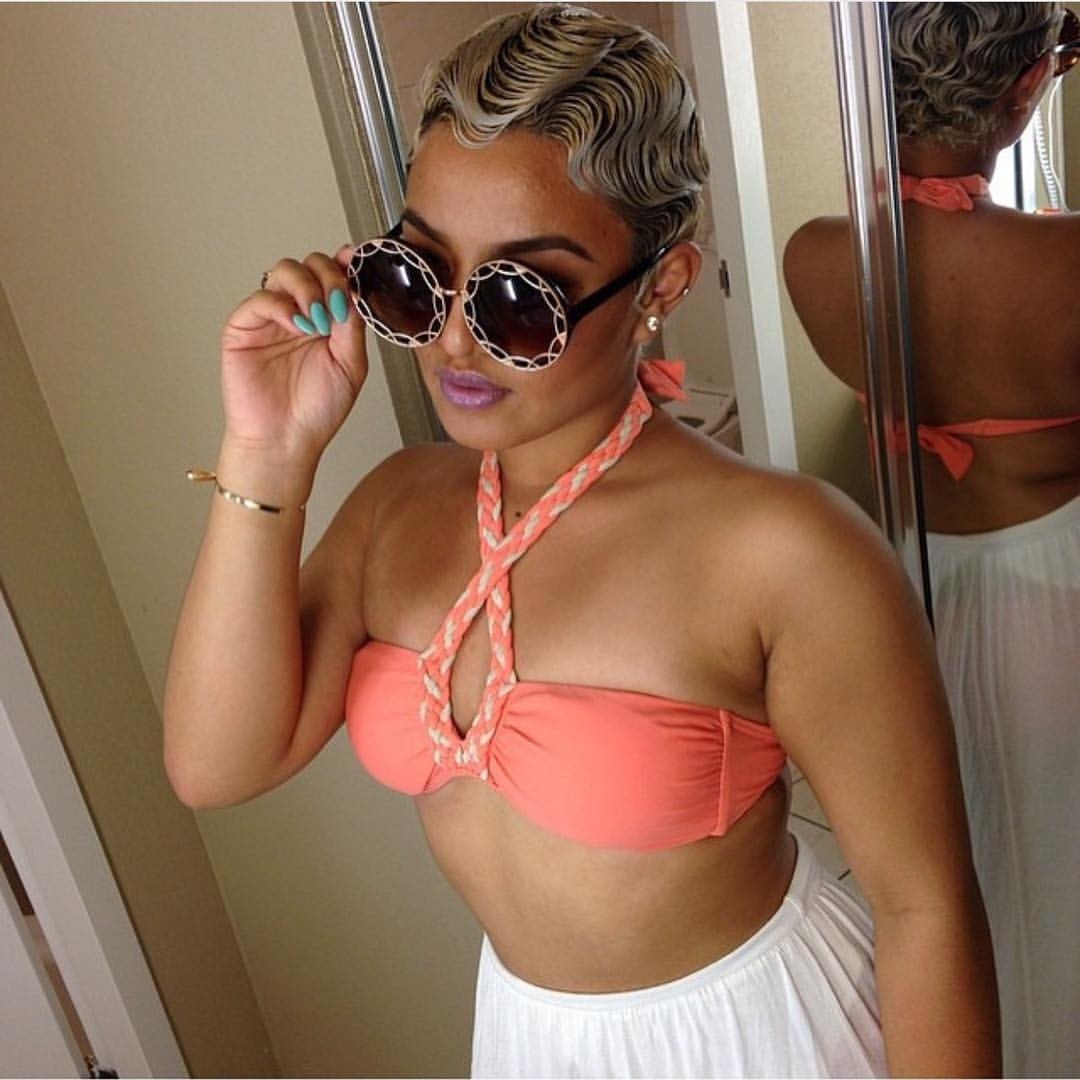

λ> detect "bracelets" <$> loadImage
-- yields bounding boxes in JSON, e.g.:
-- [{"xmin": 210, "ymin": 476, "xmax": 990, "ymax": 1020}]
[{"xmin": 183, "ymin": 469, "xmax": 308, "ymax": 515}]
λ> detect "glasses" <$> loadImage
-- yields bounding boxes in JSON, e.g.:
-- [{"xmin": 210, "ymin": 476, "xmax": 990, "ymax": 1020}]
[{"xmin": 347, "ymin": 224, "xmax": 677, "ymax": 371}]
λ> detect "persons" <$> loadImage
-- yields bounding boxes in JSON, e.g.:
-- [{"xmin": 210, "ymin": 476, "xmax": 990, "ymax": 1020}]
[
  {"xmin": 782, "ymin": 2, "xmax": 1080, "ymax": 1076},
  {"xmin": 164, "ymin": 5, "xmax": 1008, "ymax": 1080}
]
